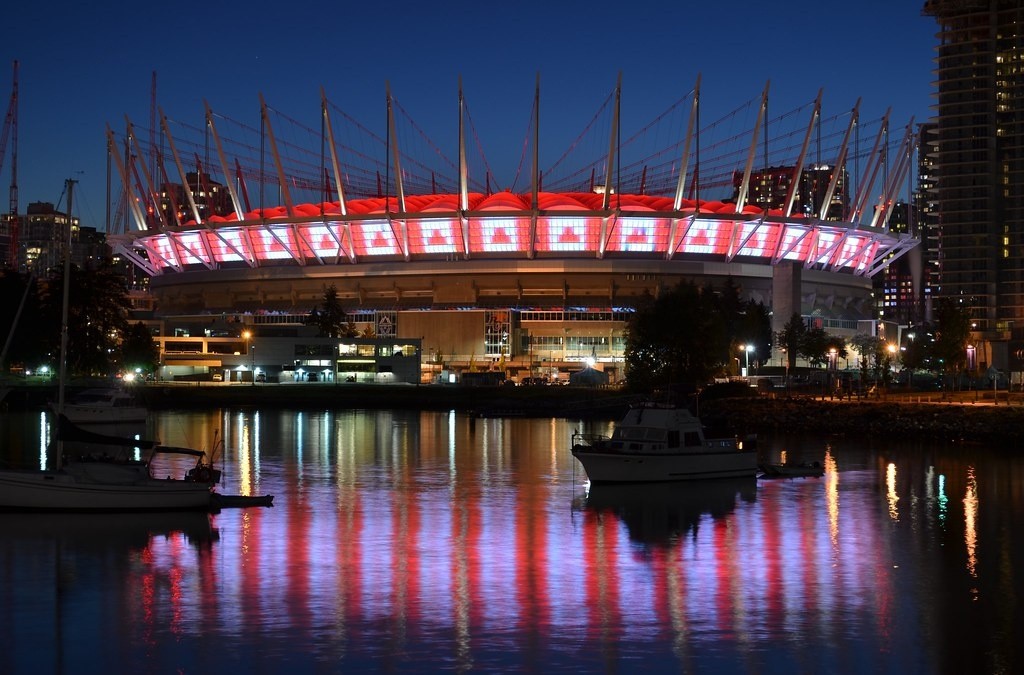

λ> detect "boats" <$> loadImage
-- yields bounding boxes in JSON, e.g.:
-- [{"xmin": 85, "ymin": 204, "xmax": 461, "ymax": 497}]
[
  {"xmin": 570, "ymin": 401, "xmax": 759, "ymax": 486},
  {"xmin": 47, "ymin": 386, "xmax": 148, "ymax": 441},
  {"xmin": 769, "ymin": 461, "xmax": 823, "ymax": 476}
]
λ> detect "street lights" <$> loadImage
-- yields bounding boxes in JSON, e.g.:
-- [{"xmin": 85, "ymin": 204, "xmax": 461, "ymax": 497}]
[
  {"xmin": 241, "ymin": 331, "xmax": 251, "ymax": 355},
  {"xmin": 738, "ymin": 343, "xmax": 756, "ymax": 369}
]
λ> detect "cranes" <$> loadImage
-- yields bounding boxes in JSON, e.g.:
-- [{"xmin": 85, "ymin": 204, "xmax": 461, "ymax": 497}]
[{"xmin": 0, "ymin": 59, "xmax": 21, "ymax": 271}]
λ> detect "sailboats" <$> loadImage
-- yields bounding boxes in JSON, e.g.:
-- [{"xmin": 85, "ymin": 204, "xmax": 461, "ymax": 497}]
[{"xmin": 0, "ymin": 174, "xmax": 224, "ymax": 514}]
